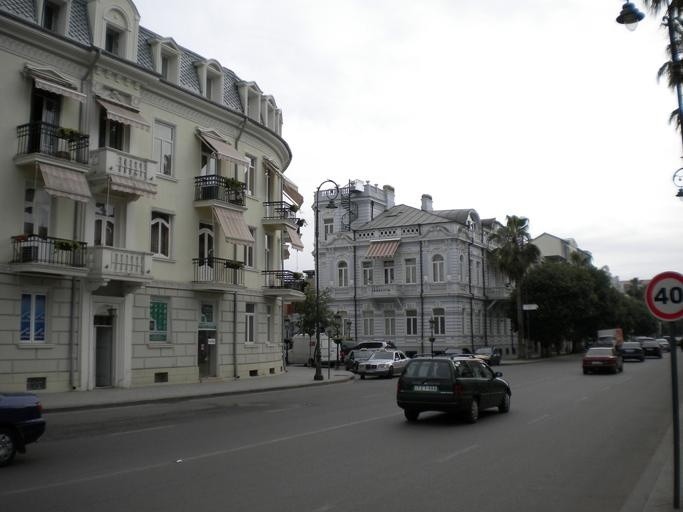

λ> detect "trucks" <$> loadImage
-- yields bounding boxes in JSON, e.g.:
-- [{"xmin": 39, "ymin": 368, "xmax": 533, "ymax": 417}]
[{"xmin": 597, "ymin": 329, "xmax": 623, "ymax": 347}]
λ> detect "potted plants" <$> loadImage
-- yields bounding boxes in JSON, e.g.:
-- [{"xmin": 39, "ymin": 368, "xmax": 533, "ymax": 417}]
[
  {"xmin": 225, "ymin": 260, "xmax": 245, "ymax": 269},
  {"xmin": 53, "ymin": 240, "xmax": 74, "ymax": 252}
]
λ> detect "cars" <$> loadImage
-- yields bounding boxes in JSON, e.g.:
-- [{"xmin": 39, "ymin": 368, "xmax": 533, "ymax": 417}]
[
  {"xmin": 624, "ymin": 335, "xmax": 683, "ymax": 362},
  {"xmin": 444, "ymin": 346, "xmax": 502, "ymax": 365},
  {"xmin": 397, "ymin": 357, "xmax": 512, "ymax": 421},
  {"xmin": 0, "ymin": 391, "xmax": 46, "ymax": 467},
  {"xmin": 344, "ymin": 340, "xmax": 411, "ymax": 379},
  {"xmin": 582, "ymin": 346, "xmax": 624, "ymax": 376}
]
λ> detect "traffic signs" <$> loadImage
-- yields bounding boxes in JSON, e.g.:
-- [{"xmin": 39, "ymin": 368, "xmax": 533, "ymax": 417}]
[{"xmin": 314, "ymin": 180, "xmax": 340, "ymax": 381}]
